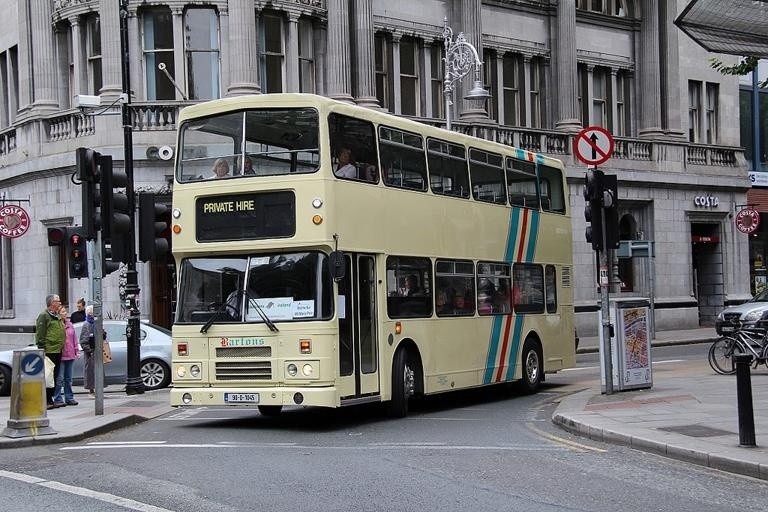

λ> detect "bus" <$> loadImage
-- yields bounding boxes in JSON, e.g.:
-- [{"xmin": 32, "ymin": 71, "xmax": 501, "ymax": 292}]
[{"xmin": 171, "ymin": 92, "xmax": 579, "ymax": 417}]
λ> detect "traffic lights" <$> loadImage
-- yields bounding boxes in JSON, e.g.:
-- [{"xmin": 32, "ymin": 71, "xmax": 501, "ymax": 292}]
[
  {"xmin": 47, "ymin": 227, "xmax": 65, "ymax": 247},
  {"xmin": 67, "ymin": 225, "xmax": 88, "ymax": 278},
  {"xmin": 583, "ymin": 169, "xmax": 619, "ymax": 251},
  {"xmin": 140, "ymin": 193, "xmax": 167, "ymax": 263},
  {"xmin": 76, "ymin": 148, "xmax": 130, "ymax": 276}
]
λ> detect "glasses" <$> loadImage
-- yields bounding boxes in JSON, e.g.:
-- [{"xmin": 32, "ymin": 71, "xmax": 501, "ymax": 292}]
[{"xmin": 53, "ymin": 300, "xmax": 61, "ymax": 303}]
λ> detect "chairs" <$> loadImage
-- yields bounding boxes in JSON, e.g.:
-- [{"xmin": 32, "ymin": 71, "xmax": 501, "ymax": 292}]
[{"xmin": 354, "ymin": 165, "xmax": 552, "ymax": 212}]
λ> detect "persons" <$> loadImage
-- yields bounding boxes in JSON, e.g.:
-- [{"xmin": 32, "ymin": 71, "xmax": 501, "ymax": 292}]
[
  {"xmin": 223, "ymin": 274, "xmax": 259, "ymax": 318},
  {"xmin": 70, "ymin": 298, "xmax": 87, "ymax": 323},
  {"xmin": 205, "ymin": 157, "xmax": 233, "ymax": 180},
  {"xmin": 331, "ymin": 147, "xmax": 354, "ymax": 180},
  {"xmin": 79, "ymin": 305, "xmax": 110, "ymax": 400},
  {"xmin": 387, "ymin": 273, "xmax": 521, "ymax": 316},
  {"xmin": 34, "ymin": 293, "xmax": 67, "ymax": 409},
  {"xmin": 232, "ymin": 154, "xmax": 257, "ymax": 176},
  {"xmin": 52, "ymin": 305, "xmax": 81, "ymax": 406}
]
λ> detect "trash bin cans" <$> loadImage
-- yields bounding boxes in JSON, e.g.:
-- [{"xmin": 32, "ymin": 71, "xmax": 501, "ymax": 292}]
[
  {"xmin": 1, "ymin": 349, "xmax": 57, "ymax": 437},
  {"xmin": 595, "ymin": 297, "xmax": 653, "ymax": 393}
]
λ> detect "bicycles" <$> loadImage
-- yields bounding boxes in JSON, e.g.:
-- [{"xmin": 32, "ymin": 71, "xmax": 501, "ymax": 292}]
[{"xmin": 708, "ymin": 317, "xmax": 768, "ymax": 375}]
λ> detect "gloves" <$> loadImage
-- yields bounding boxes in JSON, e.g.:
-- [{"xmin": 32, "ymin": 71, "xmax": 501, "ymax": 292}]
[{"xmin": 39, "ymin": 350, "xmax": 45, "ymax": 359}]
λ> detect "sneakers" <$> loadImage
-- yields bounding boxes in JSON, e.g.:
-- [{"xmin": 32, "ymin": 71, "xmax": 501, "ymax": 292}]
[
  {"xmin": 47, "ymin": 400, "xmax": 79, "ymax": 409},
  {"xmin": 88, "ymin": 394, "xmax": 95, "ymax": 400}
]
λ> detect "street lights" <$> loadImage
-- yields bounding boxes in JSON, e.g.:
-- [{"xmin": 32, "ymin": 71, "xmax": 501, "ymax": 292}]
[{"xmin": 440, "ymin": 17, "xmax": 492, "ymax": 132}]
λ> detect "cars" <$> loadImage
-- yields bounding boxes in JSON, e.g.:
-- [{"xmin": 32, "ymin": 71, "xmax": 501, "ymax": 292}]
[
  {"xmin": 715, "ymin": 288, "xmax": 768, "ymax": 343},
  {"xmin": 0, "ymin": 319, "xmax": 171, "ymax": 395}
]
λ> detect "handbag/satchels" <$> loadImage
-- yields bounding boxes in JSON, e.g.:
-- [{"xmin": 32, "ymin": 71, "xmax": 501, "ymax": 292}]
[
  {"xmin": 44, "ymin": 356, "xmax": 56, "ymax": 389},
  {"xmin": 102, "ymin": 342, "xmax": 112, "ymax": 364}
]
